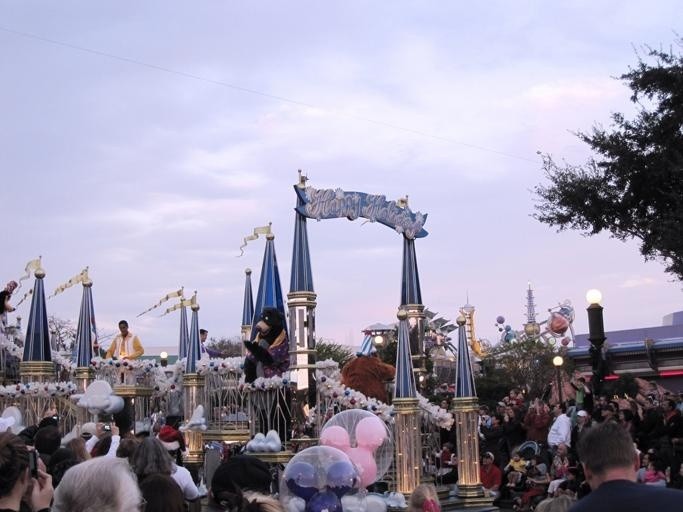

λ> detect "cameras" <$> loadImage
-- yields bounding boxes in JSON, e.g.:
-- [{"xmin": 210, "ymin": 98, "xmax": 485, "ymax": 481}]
[
  {"xmin": 52, "ymin": 414, "xmax": 59, "ymax": 419},
  {"xmin": 103, "ymin": 425, "xmax": 112, "ymax": 431}
]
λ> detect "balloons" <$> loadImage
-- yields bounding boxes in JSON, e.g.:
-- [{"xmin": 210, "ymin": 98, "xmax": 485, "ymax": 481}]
[
  {"xmin": 495, "ymin": 304, "xmax": 573, "ymax": 350},
  {"xmin": 278, "ymin": 409, "xmax": 393, "ymax": 512}
]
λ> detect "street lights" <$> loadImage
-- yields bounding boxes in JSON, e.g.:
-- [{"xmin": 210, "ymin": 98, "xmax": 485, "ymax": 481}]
[
  {"xmin": 160, "ymin": 349, "xmax": 167, "ymax": 366},
  {"xmin": 584, "ymin": 289, "xmax": 605, "ymax": 366},
  {"xmin": 372, "ymin": 336, "xmax": 383, "ymax": 358},
  {"xmin": 551, "ymin": 355, "xmax": 565, "ymax": 404}
]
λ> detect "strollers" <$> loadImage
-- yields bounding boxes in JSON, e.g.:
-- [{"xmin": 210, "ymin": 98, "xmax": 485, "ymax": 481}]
[{"xmin": 502, "ymin": 440, "xmax": 544, "ymax": 493}]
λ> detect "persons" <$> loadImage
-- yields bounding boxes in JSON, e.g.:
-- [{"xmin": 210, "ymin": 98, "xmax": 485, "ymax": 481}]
[
  {"xmin": 105, "ymin": 319, "xmax": 143, "ymax": 361},
  {"xmin": 0, "ymin": 400, "xmax": 286, "ymax": 512},
  {"xmin": 0, "ymin": 281, "xmax": 18, "ymax": 327},
  {"xmin": 407, "ymin": 358, "xmax": 682, "ymax": 512},
  {"xmin": 199, "ymin": 329, "xmax": 224, "ymax": 359}
]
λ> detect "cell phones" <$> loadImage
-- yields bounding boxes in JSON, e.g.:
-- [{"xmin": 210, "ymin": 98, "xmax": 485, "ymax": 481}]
[
  {"xmin": 481, "ymin": 416, "xmax": 486, "ymax": 422},
  {"xmin": 27, "ymin": 449, "xmax": 39, "ymax": 479}
]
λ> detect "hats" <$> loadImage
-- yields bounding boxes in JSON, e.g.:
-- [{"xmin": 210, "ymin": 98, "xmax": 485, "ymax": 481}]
[
  {"xmin": 156, "ymin": 426, "xmax": 185, "ymax": 451},
  {"xmin": 577, "ymin": 411, "xmax": 588, "ymax": 418}
]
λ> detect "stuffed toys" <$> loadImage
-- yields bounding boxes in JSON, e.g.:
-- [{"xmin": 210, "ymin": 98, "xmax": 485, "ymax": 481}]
[{"xmin": 244, "ymin": 307, "xmax": 292, "ymax": 442}]
